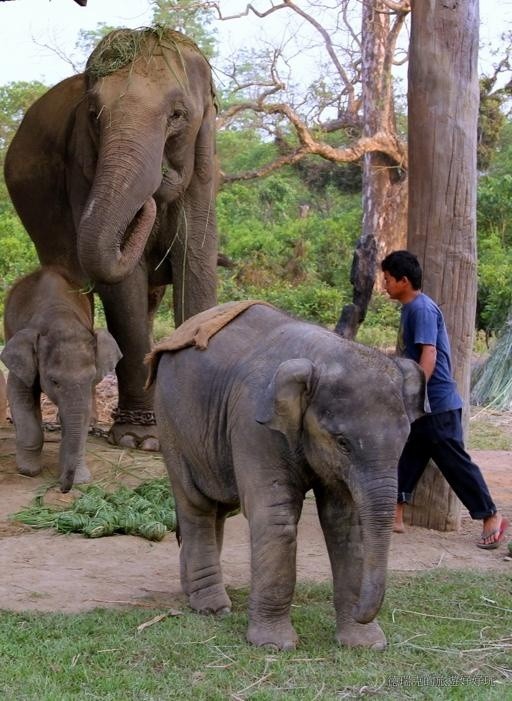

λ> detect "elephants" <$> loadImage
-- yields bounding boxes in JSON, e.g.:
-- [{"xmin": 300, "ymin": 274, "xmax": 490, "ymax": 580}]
[
  {"xmin": 151, "ymin": 300, "xmax": 432, "ymax": 654},
  {"xmin": 0, "ymin": 267, "xmax": 124, "ymax": 494},
  {"xmin": 3, "ymin": 26, "xmax": 221, "ymax": 453}
]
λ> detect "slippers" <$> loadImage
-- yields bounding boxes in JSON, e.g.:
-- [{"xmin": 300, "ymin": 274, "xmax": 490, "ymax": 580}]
[{"xmin": 476, "ymin": 515, "xmax": 509, "ymax": 550}]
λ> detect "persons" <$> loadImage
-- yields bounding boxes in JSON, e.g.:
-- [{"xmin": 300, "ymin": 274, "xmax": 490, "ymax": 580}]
[{"xmin": 379, "ymin": 249, "xmax": 507, "ymax": 550}]
